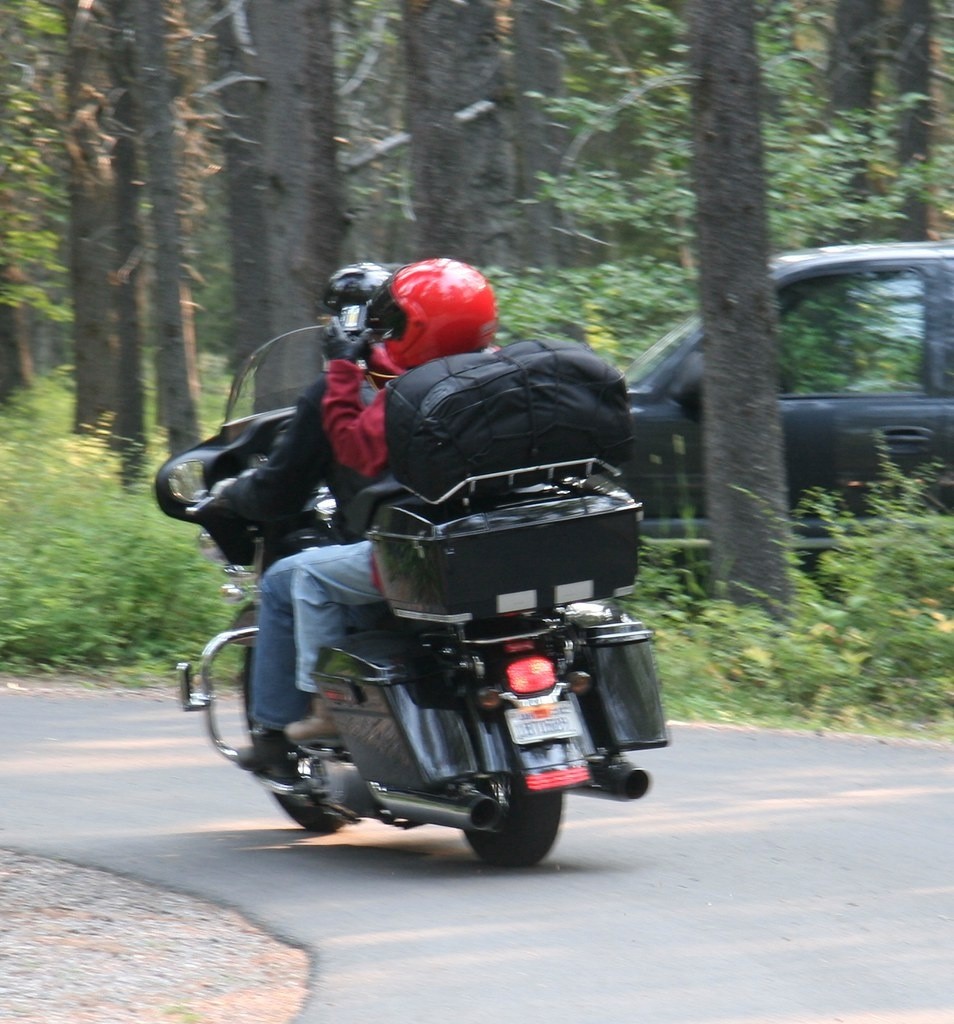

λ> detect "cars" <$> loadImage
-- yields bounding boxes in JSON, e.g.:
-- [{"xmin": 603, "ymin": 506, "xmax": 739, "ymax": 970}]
[{"xmin": 623, "ymin": 230, "xmax": 954, "ymax": 535}]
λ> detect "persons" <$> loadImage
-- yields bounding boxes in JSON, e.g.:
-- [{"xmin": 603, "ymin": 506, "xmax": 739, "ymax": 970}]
[
  {"xmin": 188, "ymin": 257, "xmax": 402, "ymax": 764},
  {"xmin": 283, "ymin": 258, "xmax": 498, "ymax": 750}
]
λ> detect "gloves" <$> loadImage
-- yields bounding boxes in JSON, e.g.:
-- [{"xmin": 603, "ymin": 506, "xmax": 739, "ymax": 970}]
[{"xmin": 315, "ymin": 313, "xmax": 374, "ymax": 362}]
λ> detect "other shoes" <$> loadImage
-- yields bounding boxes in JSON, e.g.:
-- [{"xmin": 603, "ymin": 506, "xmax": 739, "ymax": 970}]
[
  {"xmin": 281, "ymin": 691, "xmax": 352, "ymax": 751},
  {"xmin": 231, "ymin": 733, "xmax": 301, "ymax": 779}
]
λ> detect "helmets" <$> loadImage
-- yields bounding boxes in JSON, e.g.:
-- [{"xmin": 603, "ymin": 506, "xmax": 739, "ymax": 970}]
[
  {"xmin": 364, "ymin": 256, "xmax": 501, "ymax": 369},
  {"xmin": 315, "ymin": 260, "xmax": 393, "ymax": 308}
]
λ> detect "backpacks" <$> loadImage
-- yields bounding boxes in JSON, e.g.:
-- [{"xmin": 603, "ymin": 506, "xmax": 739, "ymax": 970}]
[{"xmin": 385, "ymin": 336, "xmax": 636, "ymax": 498}]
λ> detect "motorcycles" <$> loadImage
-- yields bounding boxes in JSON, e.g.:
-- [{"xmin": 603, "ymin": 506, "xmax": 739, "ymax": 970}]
[{"xmin": 154, "ymin": 323, "xmax": 669, "ymax": 871}]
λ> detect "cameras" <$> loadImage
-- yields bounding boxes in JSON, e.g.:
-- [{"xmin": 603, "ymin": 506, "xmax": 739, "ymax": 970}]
[{"xmin": 337, "ymin": 305, "xmax": 368, "ymax": 333}]
